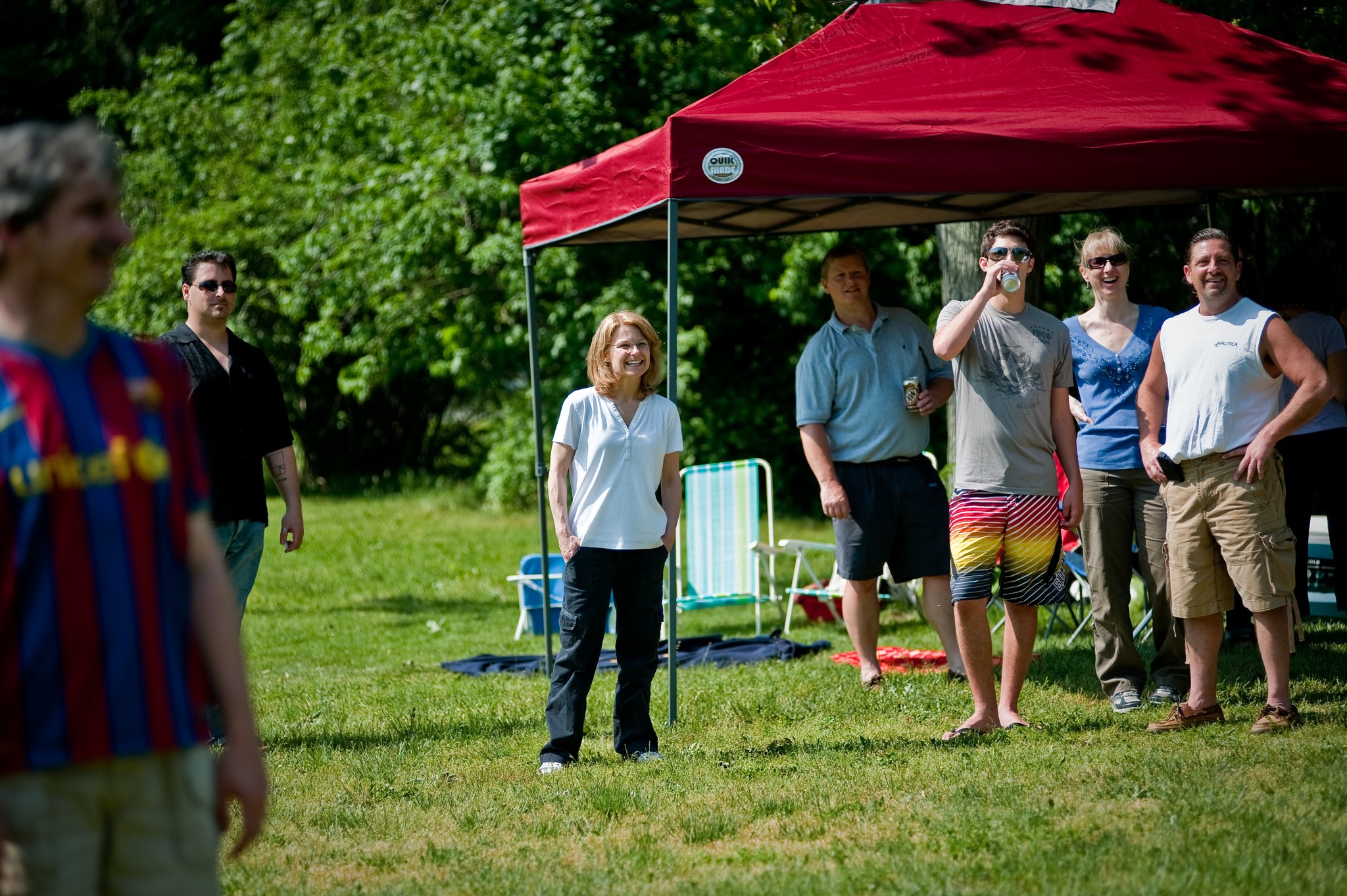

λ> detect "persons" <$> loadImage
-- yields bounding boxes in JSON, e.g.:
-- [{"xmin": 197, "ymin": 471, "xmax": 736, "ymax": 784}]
[
  {"xmin": 794, "ymin": 238, "xmax": 966, "ymax": 693},
  {"xmin": 1063, "ymin": 228, "xmax": 1193, "ymax": 712},
  {"xmin": 0, "ymin": 118, "xmax": 268, "ymax": 894},
  {"xmin": 1136, "ymin": 227, "xmax": 1338, "ymax": 732},
  {"xmin": 161, "ymin": 251, "xmax": 306, "ymax": 749},
  {"xmin": 1268, "ymin": 252, "xmax": 1347, "ymax": 621},
  {"xmin": 933, "ymin": 223, "xmax": 1085, "ymax": 743},
  {"xmin": 539, "ymin": 311, "xmax": 684, "ymax": 774}
]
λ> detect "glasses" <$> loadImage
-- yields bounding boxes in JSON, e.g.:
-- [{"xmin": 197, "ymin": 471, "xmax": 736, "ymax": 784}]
[
  {"xmin": 984, "ymin": 247, "xmax": 1033, "ymax": 263},
  {"xmin": 189, "ymin": 279, "xmax": 238, "ymax": 295},
  {"xmin": 607, "ymin": 340, "xmax": 653, "ymax": 351},
  {"xmin": 1084, "ymin": 252, "xmax": 1129, "ymax": 269}
]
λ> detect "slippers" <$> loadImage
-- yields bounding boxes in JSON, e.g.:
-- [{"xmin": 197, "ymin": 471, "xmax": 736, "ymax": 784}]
[
  {"xmin": 1003, "ymin": 721, "xmax": 1042, "ymax": 736},
  {"xmin": 944, "ymin": 727, "xmax": 998, "ymax": 745}
]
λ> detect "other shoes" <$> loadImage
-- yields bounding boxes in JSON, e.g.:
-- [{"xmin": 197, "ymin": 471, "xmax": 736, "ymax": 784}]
[
  {"xmin": 635, "ymin": 751, "xmax": 662, "ymax": 764},
  {"xmin": 948, "ymin": 669, "xmax": 967, "ymax": 681},
  {"xmin": 537, "ymin": 761, "xmax": 559, "ymax": 775},
  {"xmin": 858, "ymin": 676, "xmax": 886, "ymax": 693}
]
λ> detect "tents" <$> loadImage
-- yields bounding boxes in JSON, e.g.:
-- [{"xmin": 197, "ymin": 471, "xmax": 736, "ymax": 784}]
[{"xmin": 521, "ymin": 1, "xmax": 1346, "ymax": 725}]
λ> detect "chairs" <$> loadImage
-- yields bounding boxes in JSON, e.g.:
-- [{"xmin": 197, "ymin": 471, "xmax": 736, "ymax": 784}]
[
  {"xmin": 506, "ymin": 554, "xmax": 613, "ymax": 644},
  {"xmin": 776, "ymin": 449, "xmax": 938, "ymax": 635},
  {"xmin": 657, "ymin": 458, "xmax": 782, "ymax": 643}
]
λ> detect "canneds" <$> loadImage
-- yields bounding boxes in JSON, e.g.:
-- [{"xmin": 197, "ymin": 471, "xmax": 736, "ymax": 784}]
[
  {"xmin": 903, "ymin": 376, "xmax": 925, "ymax": 412},
  {"xmin": 1001, "ymin": 268, "xmax": 1022, "ymax": 291}
]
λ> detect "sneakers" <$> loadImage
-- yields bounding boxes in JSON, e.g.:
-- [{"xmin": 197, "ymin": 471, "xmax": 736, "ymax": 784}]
[
  {"xmin": 1250, "ymin": 704, "xmax": 1299, "ymax": 733},
  {"xmin": 1148, "ymin": 682, "xmax": 1187, "ymax": 707},
  {"xmin": 1112, "ymin": 690, "xmax": 1142, "ymax": 712},
  {"xmin": 1147, "ymin": 702, "xmax": 1224, "ymax": 734}
]
